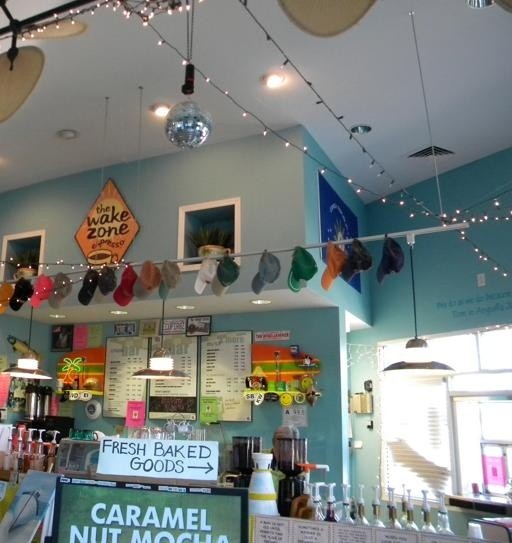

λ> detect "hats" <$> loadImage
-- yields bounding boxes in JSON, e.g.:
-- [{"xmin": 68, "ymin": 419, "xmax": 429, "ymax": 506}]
[
  {"xmin": 194, "ymin": 256, "xmax": 239, "ymax": 297},
  {"xmin": 321, "ymin": 241, "xmax": 346, "ymax": 291},
  {"xmin": 133, "ymin": 261, "xmax": 160, "ymax": 300},
  {"xmin": 97, "ymin": 267, "xmax": 116, "ymax": 294},
  {"xmin": 287, "ymin": 246, "xmax": 318, "ymax": 292},
  {"xmin": 252, "ymin": 251, "xmax": 280, "ymax": 295},
  {"xmin": 377, "ymin": 237, "xmax": 404, "ymax": 285},
  {"xmin": 77, "ymin": 267, "xmax": 98, "ymax": 305},
  {"xmin": 158, "ymin": 259, "xmax": 180, "ymax": 298},
  {"xmin": 0, "ymin": 273, "xmax": 71, "ymax": 314},
  {"xmin": 113, "ymin": 267, "xmax": 138, "ymax": 306},
  {"xmin": 342, "ymin": 239, "xmax": 372, "ymax": 283}
]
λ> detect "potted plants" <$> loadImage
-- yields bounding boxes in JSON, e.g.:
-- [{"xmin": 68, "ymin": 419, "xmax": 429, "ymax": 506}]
[
  {"xmin": 188, "ymin": 225, "xmax": 234, "ymax": 256},
  {"xmin": 13, "ymin": 248, "xmax": 38, "ymax": 279}
]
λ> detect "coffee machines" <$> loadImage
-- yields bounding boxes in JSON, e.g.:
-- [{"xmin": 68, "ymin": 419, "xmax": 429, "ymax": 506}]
[
  {"xmin": 275, "ymin": 436, "xmax": 308, "ymax": 518},
  {"xmin": 231, "ymin": 435, "xmax": 262, "ymax": 488}
]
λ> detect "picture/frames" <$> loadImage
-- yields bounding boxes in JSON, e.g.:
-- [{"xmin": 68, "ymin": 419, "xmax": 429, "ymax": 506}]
[{"xmin": 186, "ymin": 315, "xmax": 212, "ymax": 337}]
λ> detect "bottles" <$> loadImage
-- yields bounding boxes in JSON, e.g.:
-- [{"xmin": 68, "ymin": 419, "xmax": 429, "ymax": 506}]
[{"xmin": 23, "ymin": 384, "xmax": 53, "ymax": 422}]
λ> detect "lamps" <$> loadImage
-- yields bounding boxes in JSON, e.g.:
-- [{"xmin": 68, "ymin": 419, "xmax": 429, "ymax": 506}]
[
  {"xmin": 381, "ymin": 235, "xmax": 455, "ymax": 375},
  {"xmin": 132, "ymin": 300, "xmax": 190, "ymax": 379},
  {"xmin": 1, "ymin": 306, "xmax": 53, "ymax": 379}
]
